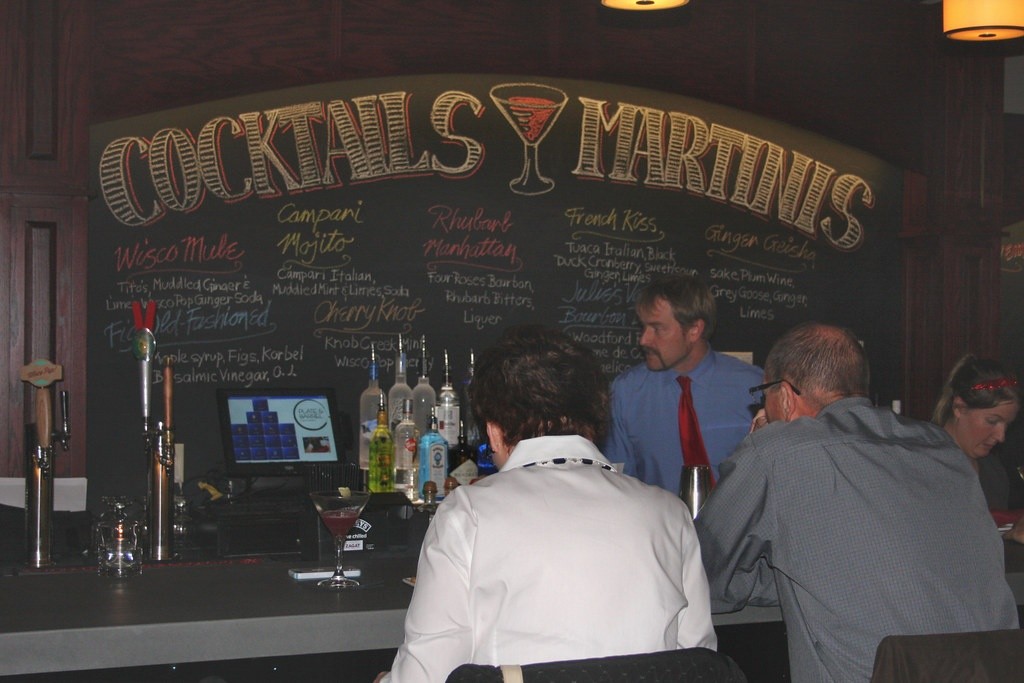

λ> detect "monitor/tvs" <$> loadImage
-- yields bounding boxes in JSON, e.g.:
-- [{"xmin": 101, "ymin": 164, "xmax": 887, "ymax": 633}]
[{"xmin": 216, "ymin": 387, "xmax": 348, "ymax": 476}]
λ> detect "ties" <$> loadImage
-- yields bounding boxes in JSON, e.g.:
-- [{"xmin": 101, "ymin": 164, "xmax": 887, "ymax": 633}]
[{"xmin": 676, "ymin": 375, "xmax": 717, "ymax": 492}]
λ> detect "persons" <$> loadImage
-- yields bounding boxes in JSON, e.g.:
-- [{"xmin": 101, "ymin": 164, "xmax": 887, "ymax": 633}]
[
  {"xmin": 694, "ymin": 321, "xmax": 1020, "ymax": 683},
  {"xmin": 603, "ymin": 272, "xmax": 765, "ymax": 498},
  {"xmin": 373, "ymin": 325, "xmax": 717, "ymax": 683},
  {"xmin": 930, "ymin": 354, "xmax": 1024, "ymax": 541}
]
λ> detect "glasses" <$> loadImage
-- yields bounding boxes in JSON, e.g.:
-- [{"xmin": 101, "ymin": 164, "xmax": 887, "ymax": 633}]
[{"xmin": 748, "ymin": 378, "xmax": 800, "ymax": 408}]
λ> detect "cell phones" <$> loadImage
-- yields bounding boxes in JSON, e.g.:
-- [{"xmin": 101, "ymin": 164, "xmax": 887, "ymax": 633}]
[{"xmin": 288, "ymin": 565, "xmax": 361, "ymax": 579}]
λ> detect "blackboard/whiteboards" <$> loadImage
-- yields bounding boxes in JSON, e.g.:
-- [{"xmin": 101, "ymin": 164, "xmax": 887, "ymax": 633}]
[{"xmin": 86, "ymin": 75, "xmax": 904, "ymax": 564}]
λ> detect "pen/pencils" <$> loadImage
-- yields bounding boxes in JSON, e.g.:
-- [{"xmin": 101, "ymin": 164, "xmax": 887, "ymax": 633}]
[{"xmin": 1017, "ymin": 467, "xmax": 1024, "ymax": 481}]
